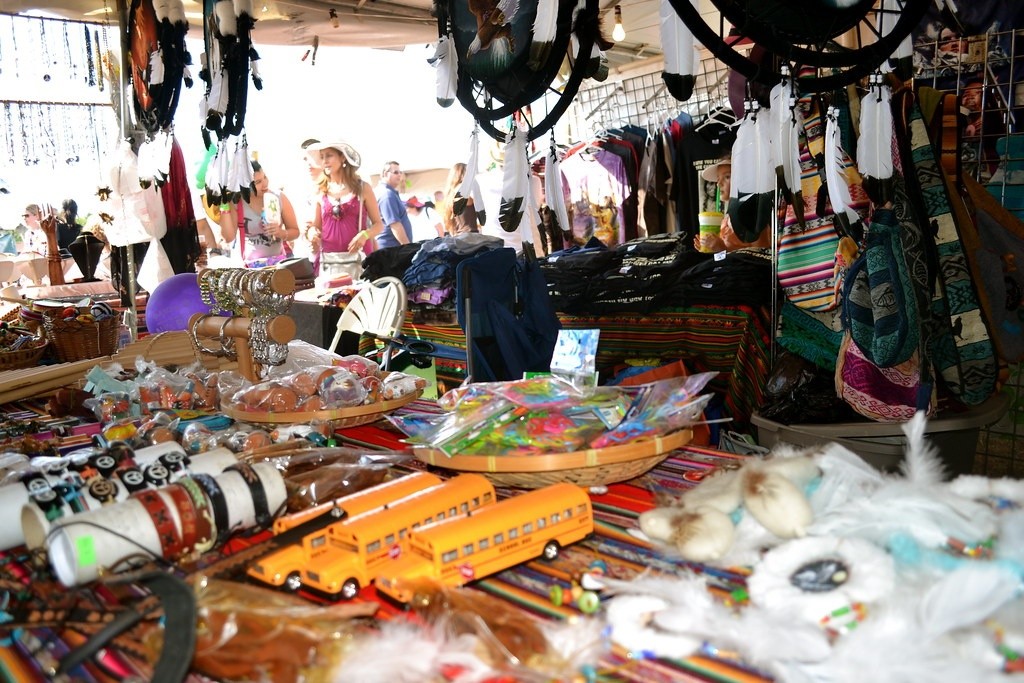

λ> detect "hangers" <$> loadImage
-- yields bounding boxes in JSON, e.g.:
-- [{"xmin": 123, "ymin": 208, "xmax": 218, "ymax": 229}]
[
  {"xmin": 645, "ymin": 86, "xmax": 680, "ymax": 147},
  {"xmin": 693, "ymin": 81, "xmax": 746, "ymax": 132},
  {"xmin": 579, "ymin": 102, "xmax": 632, "ymax": 153},
  {"xmin": 483, "ymin": 140, "xmax": 505, "ymax": 170}
]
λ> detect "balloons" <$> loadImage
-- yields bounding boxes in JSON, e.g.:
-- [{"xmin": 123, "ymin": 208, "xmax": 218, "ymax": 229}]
[{"xmin": 145, "ymin": 272, "xmax": 234, "ymax": 336}]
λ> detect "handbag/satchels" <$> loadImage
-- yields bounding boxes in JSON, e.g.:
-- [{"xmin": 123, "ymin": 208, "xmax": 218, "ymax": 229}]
[{"xmin": 319, "ymin": 250, "xmax": 360, "ymax": 280}]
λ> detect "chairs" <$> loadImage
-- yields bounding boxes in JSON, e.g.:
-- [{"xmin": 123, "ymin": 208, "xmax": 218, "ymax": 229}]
[{"xmin": 0, "ymin": 258, "xmax": 46, "ymax": 288}]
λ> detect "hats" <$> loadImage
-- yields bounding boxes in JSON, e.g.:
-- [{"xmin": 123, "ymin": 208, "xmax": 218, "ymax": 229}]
[
  {"xmin": 701, "ymin": 159, "xmax": 731, "ymax": 183},
  {"xmin": 305, "ymin": 135, "xmax": 361, "ymax": 172}
]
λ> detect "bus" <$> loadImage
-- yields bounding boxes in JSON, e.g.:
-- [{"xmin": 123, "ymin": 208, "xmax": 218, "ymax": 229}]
[
  {"xmin": 376, "ymin": 479, "xmax": 596, "ymax": 609},
  {"xmin": 302, "ymin": 470, "xmax": 497, "ymax": 601},
  {"xmin": 251, "ymin": 471, "xmax": 446, "ymax": 593}
]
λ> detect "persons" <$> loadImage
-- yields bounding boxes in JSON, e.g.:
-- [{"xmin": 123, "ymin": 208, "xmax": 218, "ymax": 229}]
[
  {"xmin": 37, "ymin": 203, "xmax": 65, "ymax": 286},
  {"xmin": 22, "ymin": 204, "xmax": 48, "ymax": 258},
  {"xmin": 692, "ymin": 152, "xmax": 773, "ymax": 253},
  {"xmin": 191, "ymin": 138, "xmax": 481, "ymax": 284},
  {"xmin": 53, "ymin": 199, "xmax": 84, "ymax": 258}
]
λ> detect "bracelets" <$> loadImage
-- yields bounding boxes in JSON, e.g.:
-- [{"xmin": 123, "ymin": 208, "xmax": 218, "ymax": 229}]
[
  {"xmin": 221, "ymin": 211, "xmax": 231, "ymax": 214},
  {"xmin": 220, "ymin": 204, "xmax": 230, "ymax": 211},
  {"xmin": 361, "ymin": 230, "xmax": 369, "ymax": 239},
  {"xmin": 139, "ymin": 462, "xmax": 272, "ymax": 560},
  {"xmin": 48, "ymin": 254, "xmax": 62, "ymax": 262},
  {"xmin": 284, "ymin": 230, "xmax": 288, "ymax": 241},
  {"xmin": 195, "ymin": 267, "xmax": 294, "ymax": 367}
]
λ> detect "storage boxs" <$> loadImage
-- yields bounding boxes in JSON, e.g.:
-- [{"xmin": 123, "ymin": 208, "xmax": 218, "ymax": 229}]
[{"xmin": 751, "ymin": 390, "xmax": 1012, "ymax": 476}]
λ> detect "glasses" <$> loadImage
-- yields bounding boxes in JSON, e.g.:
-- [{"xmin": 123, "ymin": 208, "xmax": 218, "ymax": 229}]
[
  {"xmin": 389, "ymin": 170, "xmax": 402, "ymax": 175},
  {"xmin": 22, "ymin": 214, "xmax": 29, "ymax": 218},
  {"xmin": 333, "ymin": 197, "xmax": 340, "ymax": 215}
]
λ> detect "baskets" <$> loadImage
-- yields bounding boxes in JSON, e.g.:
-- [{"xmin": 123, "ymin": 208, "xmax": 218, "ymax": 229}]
[
  {"xmin": 220, "ymin": 370, "xmax": 424, "ymax": 430},
  {"xmin": 0, "ymin": 329, "xmax": 50, "ymax": 370},
  {"xmin": 412, "ymin": 424, "xmax": 693, "ymax": 490},
  {"xmin": 42, "ymin": 308, "xmax": 121, "ymax": 363}
]
